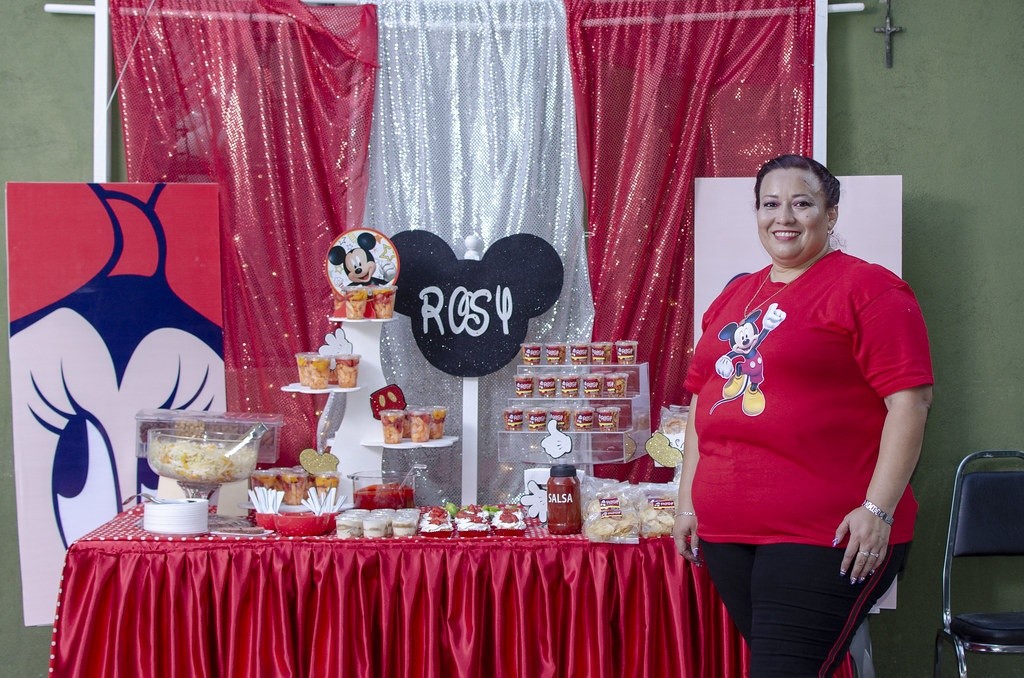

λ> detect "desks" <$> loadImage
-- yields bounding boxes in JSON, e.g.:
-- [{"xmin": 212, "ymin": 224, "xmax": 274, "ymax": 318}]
[{"xmin": 45, "ymin": 500, "xmax": 876, "ymax": 678}]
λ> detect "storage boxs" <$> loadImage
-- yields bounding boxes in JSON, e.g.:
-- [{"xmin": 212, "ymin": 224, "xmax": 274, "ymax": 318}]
[
  {"xmin": 134, "ymin": 409, "xmax": 284, "ymax": 463},
  {"xmin": 156, "ymin": 475, "xmax": 252, "ymax": 515}
]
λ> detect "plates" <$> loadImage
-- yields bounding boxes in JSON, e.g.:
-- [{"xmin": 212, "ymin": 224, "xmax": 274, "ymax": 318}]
[
  {"xmin": 418, "ymin": 528, "xmax": 526, "ymax": 536},
  {"xmin": 143, "ymin": 499, "xmax": 209, "ymax": 533},
  {"xmin": 210, "ymin": 530, "xmax": 274, "ymax": 536}
]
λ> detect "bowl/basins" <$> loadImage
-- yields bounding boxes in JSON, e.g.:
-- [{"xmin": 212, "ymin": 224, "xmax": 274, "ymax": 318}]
[
  {"xmin": 147, "ymin": 429, "xmax": 262, "ymax": 483},
  {"xmin": 347, "ymin": 470, "xmax": 420, "ymax": 512},
  {"xmin": 254, "ymin": 511, "xmax": 340, "ymax": 536}
]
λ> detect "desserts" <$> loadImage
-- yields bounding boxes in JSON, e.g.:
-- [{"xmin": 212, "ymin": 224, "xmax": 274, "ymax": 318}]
[{"xmin": 419, "ymin": 503, "xmax": 528, "ymax": 537}]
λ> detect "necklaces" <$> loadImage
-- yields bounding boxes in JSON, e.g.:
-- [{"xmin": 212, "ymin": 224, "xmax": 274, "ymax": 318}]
[{"xmin": 739, "ymin": 244, "xmax": 830, "ymax": 325}]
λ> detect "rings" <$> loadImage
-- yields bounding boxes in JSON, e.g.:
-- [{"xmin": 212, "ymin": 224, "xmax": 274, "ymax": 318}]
[
  {"xmin": 860, "ymin": 551, "xmax": 869, "ymax": 557},
  {"xmin": 866, "ymin": 564, "xmax": 874, "ymax": 575},
  {"xmin": 870, "ymin": 553, "xmax": 879, "ymax": 558}
]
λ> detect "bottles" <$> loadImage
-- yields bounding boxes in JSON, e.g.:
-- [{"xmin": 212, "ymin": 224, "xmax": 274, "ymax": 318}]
[{"xmin": 547, "ymin": 465, "xmax": 580, "ymax": 534}]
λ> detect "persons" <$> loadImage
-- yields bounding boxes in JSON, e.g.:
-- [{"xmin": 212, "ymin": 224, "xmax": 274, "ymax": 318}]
[{"xmin": 672, "ymin": 154, "xmax": 934, "ymax": 678}]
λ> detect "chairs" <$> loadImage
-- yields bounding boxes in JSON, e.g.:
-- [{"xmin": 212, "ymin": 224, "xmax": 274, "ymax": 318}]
[{"xmin": 932, "ymin": 449, "xmax": 1024, "ymax": 678}]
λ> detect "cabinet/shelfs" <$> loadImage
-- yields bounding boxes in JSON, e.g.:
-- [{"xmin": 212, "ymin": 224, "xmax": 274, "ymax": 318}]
[{"xmin": 496, "ymin": 362, "xmax": 653, "ymax": 463}]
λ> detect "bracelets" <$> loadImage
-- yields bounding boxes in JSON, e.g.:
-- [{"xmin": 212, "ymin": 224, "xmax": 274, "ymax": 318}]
[
  {"xmin": 674, "ymin": 512, "xmax": 695, "ymax": 517},
  {"xmin": 862, "ymin": 500, "xmax": 894, "ymax": 525}
]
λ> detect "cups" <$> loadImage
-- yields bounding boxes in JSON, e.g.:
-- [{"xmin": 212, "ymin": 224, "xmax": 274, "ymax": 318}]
[
  {"xmin": 522, "ymin": 341, "xmax": 637, "ymax": 365},
  {"xmin": 295, "ymin": 351, "xmax": 361, "ymax": 390},
  {"xmin": 513, "ymin": 373, "xmax": 628, "ymax": 398},
  {"xmin": 343, "ymin": 286, "xmax": 398, "ymax": 320},
  {"xmin": 379, "ymin": 404, "xmax": 449, "ymax": 444},
  {"xmin": 596, "ymin": 406, "xmax": 621, "ymax": 431},
  {"xmin": 251, "ymin": 466, "xmax": 340, "ymax": 505},
  {"xmin": 503, "ymin": 407, "xmax": 595, "ymax": 432},
  {"xmin": 335, "ymin": 509, "xmax": 420, "ymax": 538}
]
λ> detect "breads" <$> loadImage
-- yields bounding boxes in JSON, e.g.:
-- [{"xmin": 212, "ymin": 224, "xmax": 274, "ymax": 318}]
[{"xmin": 580, "ymin": 494, "xmax": 679, "ymax": 538}]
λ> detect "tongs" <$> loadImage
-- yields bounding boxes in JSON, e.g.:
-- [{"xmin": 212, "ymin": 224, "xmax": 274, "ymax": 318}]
[{"xmin": 224, "ymin": 422, "xmax": 269, "ymax": 457}]
